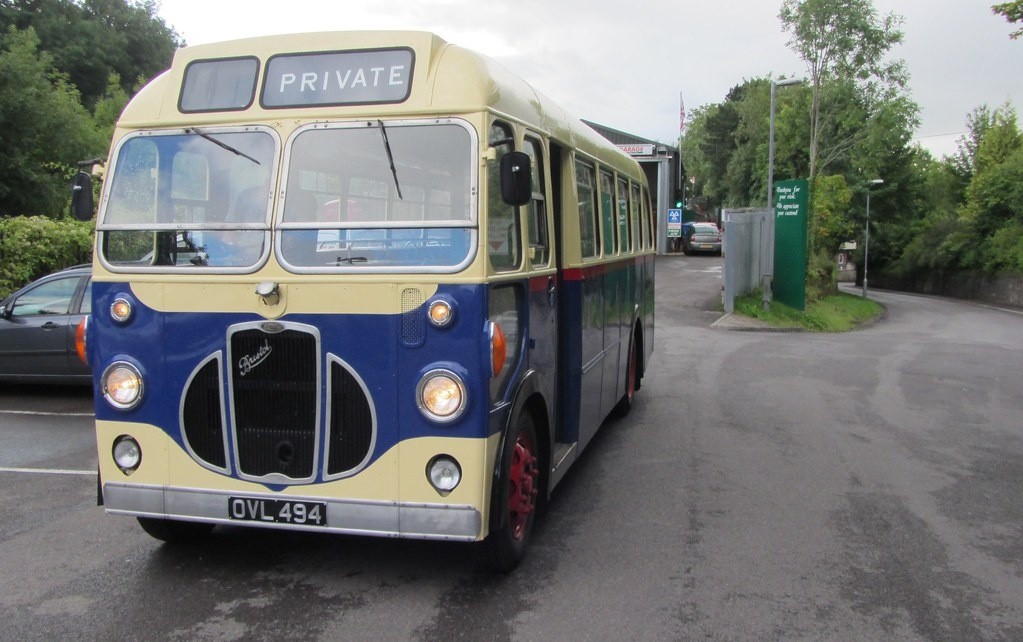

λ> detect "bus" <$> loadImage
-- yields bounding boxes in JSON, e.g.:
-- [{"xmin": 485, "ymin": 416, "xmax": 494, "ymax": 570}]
[{"xmin": 71, "ymin": 30, "xmax": 657, "ymax": 566}]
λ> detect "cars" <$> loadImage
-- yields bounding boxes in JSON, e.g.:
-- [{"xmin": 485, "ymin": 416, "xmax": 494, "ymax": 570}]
[
  {"xmin": 1, "ymin": 261, "xmax": 92, "ymax": 391},
  {"xmin": 681, "ymin": 223, "xmax": 722, "ymax": 256}
]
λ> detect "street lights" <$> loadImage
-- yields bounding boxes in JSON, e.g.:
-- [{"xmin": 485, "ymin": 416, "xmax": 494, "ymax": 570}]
[
  {"xmin": 862, "ymin": 179, "xmax": 885, "ymax": 299},
  {"xmin": 764, "ymin": 80, "xmax": 805, "ymax": 305}
]
